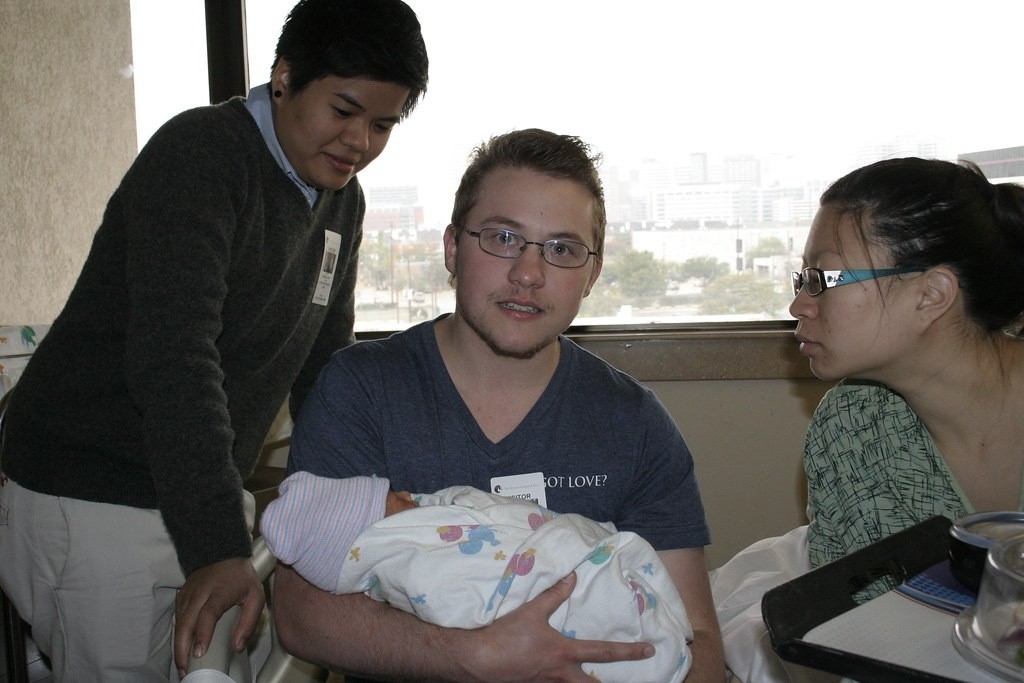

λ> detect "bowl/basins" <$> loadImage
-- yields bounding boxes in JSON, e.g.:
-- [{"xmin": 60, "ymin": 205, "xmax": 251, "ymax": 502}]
[{"xmin": 949, "ymin": 511, "xmax": 1024, "ymax": 595}]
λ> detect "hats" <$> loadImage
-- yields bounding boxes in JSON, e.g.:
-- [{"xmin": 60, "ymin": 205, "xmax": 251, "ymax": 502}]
[{"xmin": 261, "ymin": 469, "xmax": 389, "ymax": 592}]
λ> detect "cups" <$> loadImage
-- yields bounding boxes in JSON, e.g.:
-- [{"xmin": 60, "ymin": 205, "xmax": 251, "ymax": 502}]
[{"xmin": 951, "ymin": 532, "xmax": 1024, "ymax": 683}]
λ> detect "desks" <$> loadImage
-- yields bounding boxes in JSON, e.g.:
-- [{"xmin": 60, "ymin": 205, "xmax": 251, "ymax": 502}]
[{"xmin": 760, "ymin": 514, "xmax": 1023, "ymax": 683}]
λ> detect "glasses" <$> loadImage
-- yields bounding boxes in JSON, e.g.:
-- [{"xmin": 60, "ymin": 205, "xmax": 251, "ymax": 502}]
[
  {"xmin": 456, "ymin": 223, "xmax": 598, "ymax": 269},
  {"xmin": 791, "ymin": 266, "xmax": 925, "ymax": 297}
]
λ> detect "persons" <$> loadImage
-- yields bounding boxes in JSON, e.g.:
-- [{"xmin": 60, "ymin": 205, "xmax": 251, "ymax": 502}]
[
  {"xmin": 790, "ymin": 155, "xmax": 1023, "ymax": 606},
  {"xmin": 0, "ymin": 0, "xmax": 430, "ymax": 683},
  {"xmin": 273, "ymin": 126, "xmax": 728, "ymax": 683},
  {"xmin": 262, "ymin": 468, "xmax": 693, "ymax": 683}
]
